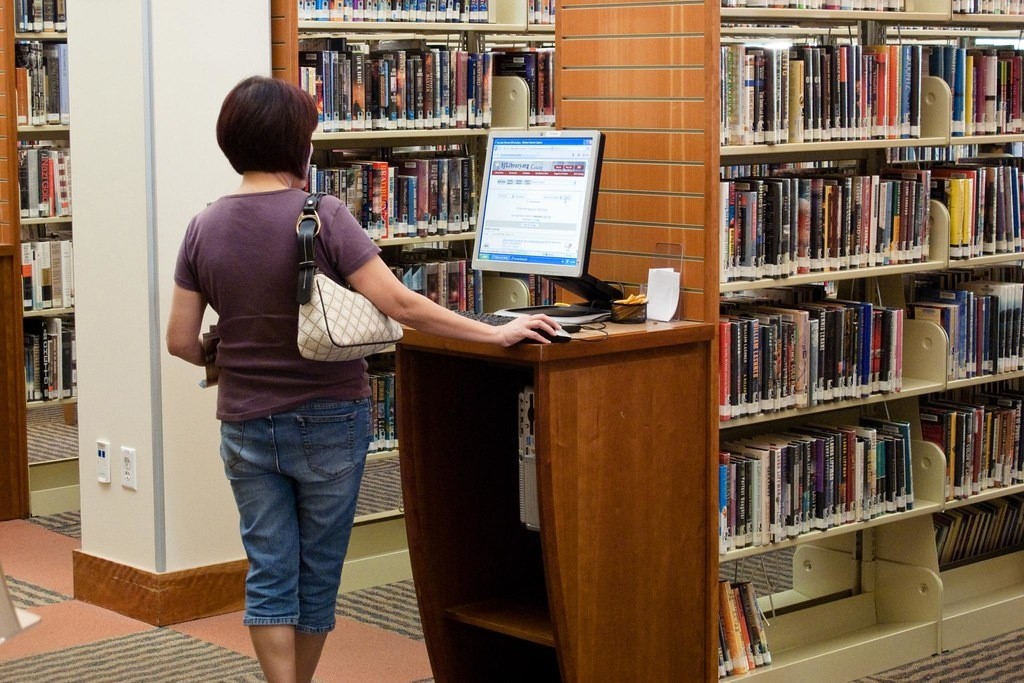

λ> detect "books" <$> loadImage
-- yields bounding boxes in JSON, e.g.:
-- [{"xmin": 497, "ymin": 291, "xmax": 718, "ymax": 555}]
[
  {"xmin": 13, "ymin": 0, "xmax": 78, "ymax": 402},
  {"xmin": 718, "ymin": 0, "xmax": 1024, "ymax": 680},
  {"xmin": 295, "ymin": 1, "xmax": 558, "ymax": 442}
]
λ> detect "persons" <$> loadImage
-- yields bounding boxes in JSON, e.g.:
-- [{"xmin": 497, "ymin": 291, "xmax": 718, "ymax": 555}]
[{"xmin": 166, "ymin": 75, "xmax": 562, "ymax": 683}]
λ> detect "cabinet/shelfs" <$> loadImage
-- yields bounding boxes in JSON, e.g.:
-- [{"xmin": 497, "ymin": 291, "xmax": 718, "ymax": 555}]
[
  {"xmin": 268, "ymin": 0, "xmax": 1024, "ymax": 683},
  {"xmin": 0, "ymin": 0, "xmax": 80, "ymax": 521}
]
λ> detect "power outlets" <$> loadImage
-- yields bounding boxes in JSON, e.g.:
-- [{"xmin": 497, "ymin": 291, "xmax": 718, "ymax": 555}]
[{"xmin": 119, "ymin": 446, "xmax": 137, "ymax": 491}]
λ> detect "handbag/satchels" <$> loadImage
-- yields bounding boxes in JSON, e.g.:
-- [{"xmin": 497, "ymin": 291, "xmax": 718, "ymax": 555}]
[{"xmin": 295, "ymin": 190, "xmax": 404, "ymax": 361}]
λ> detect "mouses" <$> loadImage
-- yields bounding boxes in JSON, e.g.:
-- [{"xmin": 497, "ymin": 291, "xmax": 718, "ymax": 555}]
[{"xmin": 521, "ymin": 326, "xmax": 571, "ymax": 343}]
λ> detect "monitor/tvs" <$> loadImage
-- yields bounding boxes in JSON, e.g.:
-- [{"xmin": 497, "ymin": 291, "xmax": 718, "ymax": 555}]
[{"xmin": 473, "ymin": 127, "xmax": 625, "ymax": 313}]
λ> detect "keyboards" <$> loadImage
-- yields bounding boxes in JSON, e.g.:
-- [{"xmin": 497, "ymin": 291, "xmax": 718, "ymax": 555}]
[{"xmin": 452, "ymin": 308, "xmax": 581, "ymax": 334}]
